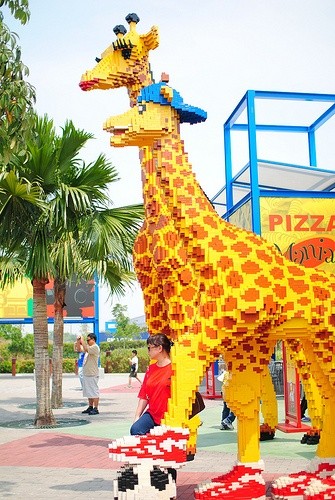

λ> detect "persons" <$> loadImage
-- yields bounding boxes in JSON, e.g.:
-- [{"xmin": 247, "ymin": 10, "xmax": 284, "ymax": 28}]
[
  {"xmin": 73, "ymin": 342, "xmax": 85, "ymax": 390},
  {"xmin": 220, "ymin": 403, "xmax": 235, "ymax": 431},
  {"xmin": 125, "ymin": 350, "xmax": 142, "ymax": 389},
  {"xmin": 131, "ymin": 333, "xmax": 173, "ymax": 435},
  {"xmin": 76, "ymin": 333, "xmax": 100, "ymax": 414},
  {"xmin": 300, "ymin": 397, "xmax": 310, "ymax": 422}
]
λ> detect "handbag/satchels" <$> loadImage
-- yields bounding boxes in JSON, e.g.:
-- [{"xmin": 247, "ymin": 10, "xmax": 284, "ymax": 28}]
[{"xmin": 189, "ymin": 392, "xmax": 205, "ymax": 420}]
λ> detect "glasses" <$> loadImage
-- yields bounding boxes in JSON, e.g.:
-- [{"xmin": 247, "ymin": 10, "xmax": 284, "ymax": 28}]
[
  {"xmin": 148, "ymin": 345, "xmax": 157, "ymax": 351},
  {"xmin": 86, "ymin": 338, "xmax": 92, "ymax": 342}
]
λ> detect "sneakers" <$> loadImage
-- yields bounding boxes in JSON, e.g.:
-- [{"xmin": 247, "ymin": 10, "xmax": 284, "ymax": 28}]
[
  {"xmin": 223, "ymin": 418, "xmax": 234, "ymax": 429},
  {"xmin": 220, "ymin": 421, "xmax": 227, "ymax": 430}
]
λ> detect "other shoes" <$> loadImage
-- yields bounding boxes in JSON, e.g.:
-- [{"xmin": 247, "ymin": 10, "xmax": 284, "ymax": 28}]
[
  {"xmin": 126, "ymin": 385, "xmax": 132, "ymax": 389},
  {"xmin": 300, "ymin": 418, "xmax": 309, "ymax": 422},
  {"xmin": 82, "ymin": 408, "xmax": 93, "ymax": 413},
  {"xmin": 89, "ymin": 409, "xmax": 99, "ymax": 415}
]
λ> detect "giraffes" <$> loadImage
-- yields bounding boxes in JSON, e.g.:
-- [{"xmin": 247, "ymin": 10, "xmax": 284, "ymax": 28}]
[{"xmin": 80, "ymin": 12, "xmax": 334, "ymax": 500}]
